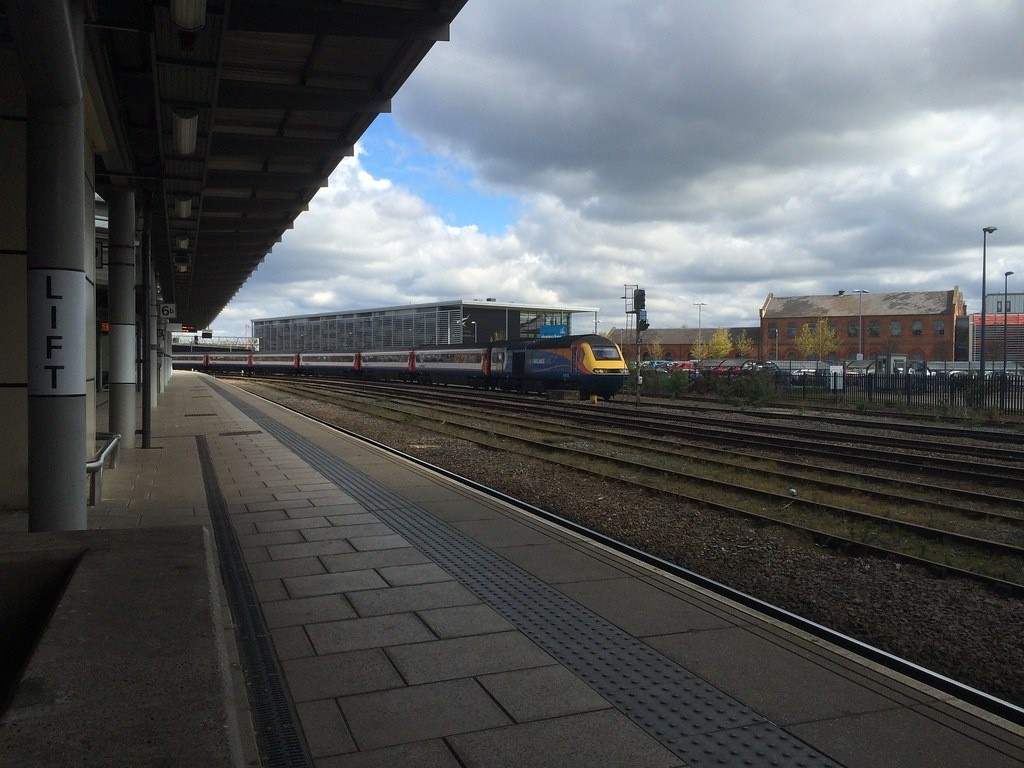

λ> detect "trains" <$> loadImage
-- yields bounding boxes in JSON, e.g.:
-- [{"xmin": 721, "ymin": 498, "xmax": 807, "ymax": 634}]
[{"xmin": 172, "ymin": 333, "xmax": 630, "ymax": 399}]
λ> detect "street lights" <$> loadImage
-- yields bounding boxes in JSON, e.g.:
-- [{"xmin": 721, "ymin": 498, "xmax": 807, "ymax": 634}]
[
  {"xmin": 693, "ymin": 302, "xmax": 708, "ymax": 361},
  {"xmin": 621, "ymin": 328, "xmax": 624, "ymax": 354},
  {"xmin": 593, "ymin": 320, "xmax": 602, "ymax": 334},
  {"xmin": 818, "ymin": 320, "xmax": 823, "ymax": 361},
  {"xmin": 852, "ymin": 290, "xmax": 870, "ymax": 360},
  {"xmin": 980, "ymin": 226, "xmax": 999, "ymax": 405},
  {"xmin": 471, "ymin": 321, "xmax": 477, "ymax": 343},
  {"xmin": 1003, "ymin": 271, "xmax": 1014, "ymax": 397},
  {"xmin": 775, "ymin": 329, "xmax": 779, "ymax": 360}
]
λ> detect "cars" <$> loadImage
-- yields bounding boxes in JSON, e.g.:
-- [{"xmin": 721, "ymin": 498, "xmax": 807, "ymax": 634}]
[
  {"xmin": 634, "ymin": 360, "xmax": 859, "ymax": 383},
  {"xmin": 859, "ymin": 368, "xmax": 1021, "ymax": 385}
]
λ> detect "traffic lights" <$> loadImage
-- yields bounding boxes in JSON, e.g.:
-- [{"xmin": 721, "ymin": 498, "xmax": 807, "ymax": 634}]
[
  {"xmin": 639, "ymin": 321, "xmax": 650, "ymax": 331},
  {"xmin": 634, "ymin": 289, "xmax": 646, "ymax": 310}
]
